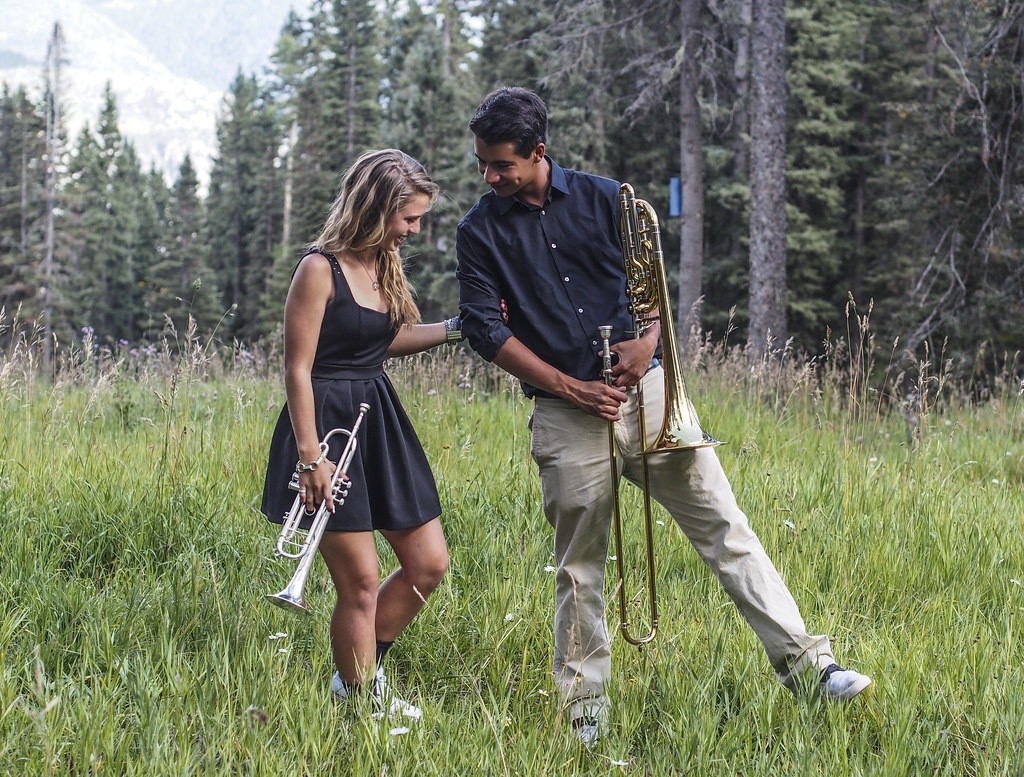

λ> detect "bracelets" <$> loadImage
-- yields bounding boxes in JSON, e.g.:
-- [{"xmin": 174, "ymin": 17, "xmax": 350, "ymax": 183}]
[
  {"xmin": 296, "ymin": 442, "xmax": 329, "ymax": 473},
  {"xmin": 444, "ymin": 316, "xmax": 466, "ymax": 346}
]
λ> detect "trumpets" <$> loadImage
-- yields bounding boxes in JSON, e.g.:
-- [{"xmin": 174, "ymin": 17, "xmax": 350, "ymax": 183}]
[{"xmin": 263, "ymin": 401, "xmax": 372, "ymax": 617}]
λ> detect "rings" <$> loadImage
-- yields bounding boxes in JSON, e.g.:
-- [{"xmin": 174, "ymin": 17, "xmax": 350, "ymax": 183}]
[{"xmin": 299, "ymin": 489, "xmax": 307, "ymax": 493}]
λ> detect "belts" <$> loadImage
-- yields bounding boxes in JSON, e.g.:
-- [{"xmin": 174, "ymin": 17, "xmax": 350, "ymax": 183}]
[{"xmin": 534, "ymin": 358, "xmax": 659, "ymax": 399}]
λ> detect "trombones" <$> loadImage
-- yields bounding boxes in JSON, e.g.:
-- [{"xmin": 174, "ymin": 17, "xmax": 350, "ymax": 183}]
[{"xmin": 594, "ymin": 181, "xmax": 733, "ymax": 647}]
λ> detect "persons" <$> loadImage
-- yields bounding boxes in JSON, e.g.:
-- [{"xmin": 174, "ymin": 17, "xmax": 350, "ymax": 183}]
[
  {"xmin": 455, "ymin": 86, "xmax": 872, "ymax": 751},
  {"xmin": 260, "ymin": 148, "xmax": 508, "ymax": 736}
]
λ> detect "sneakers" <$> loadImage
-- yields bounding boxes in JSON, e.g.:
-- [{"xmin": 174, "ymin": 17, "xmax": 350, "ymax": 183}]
[
  {"xmin": 793, "ymin": 664, "xmax": 872, "ymax": 711},
  {"xmin": 572, "ymin": 716, "xmax": 609, "ymax": 750},
  {"xmin": 330, "ymin": 670, "xmax": 423, "ymax": 724},
  {"xmin": 341, "ymin": 712, "xmax": 404, "ymax": 756}
]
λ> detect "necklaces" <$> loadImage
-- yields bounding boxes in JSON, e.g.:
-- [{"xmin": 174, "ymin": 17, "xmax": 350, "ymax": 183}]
[{"xmin": 355, "ymin": 252, "xmax": 382, "ymax": 290}]
